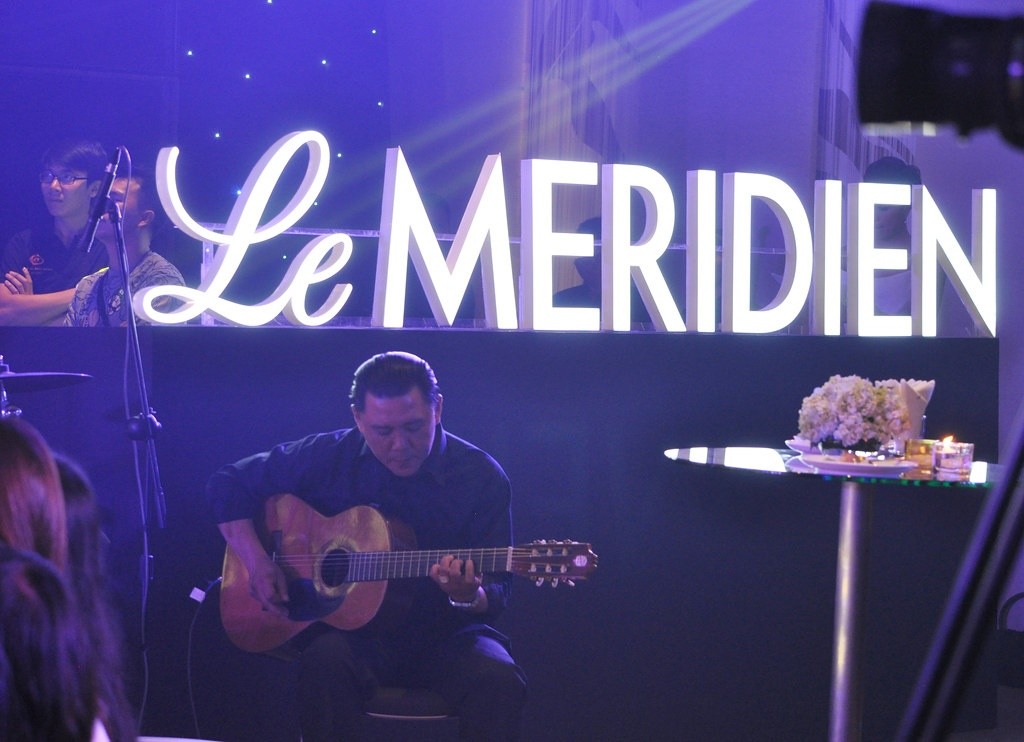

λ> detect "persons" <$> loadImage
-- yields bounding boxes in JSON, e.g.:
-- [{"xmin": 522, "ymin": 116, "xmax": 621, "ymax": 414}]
[
  {"xmin": 552, "ymin": 215, "xmax": 656, "ymax": 324},
  {"xmin": 62, "ymin": 175, "xmax": 186, "ymax": 326},
  {"xmin": 0, "ymin": 417, "xmax": 141, "ymax": 742},
  {"xmin": 840, "ymin": 157, "xmax": 946, "ymax": 338},
  {"xmin": 0, "ymin": 133, "xmax": 109, "ymax": 326},
  {"xmin": 203, "ymin": 352, "xmax": 527, "ymax": 742}
]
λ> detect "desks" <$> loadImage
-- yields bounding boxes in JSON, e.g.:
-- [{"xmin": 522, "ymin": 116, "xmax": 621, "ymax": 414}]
[{"xmin": 665, "ymin": 446, "xmax": 1000, "ymax": 742}]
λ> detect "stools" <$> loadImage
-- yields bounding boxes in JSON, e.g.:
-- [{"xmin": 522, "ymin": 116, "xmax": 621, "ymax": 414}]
[{"xmin": 365, "ymin": 688, "xmax": 459, "ymax": 721}]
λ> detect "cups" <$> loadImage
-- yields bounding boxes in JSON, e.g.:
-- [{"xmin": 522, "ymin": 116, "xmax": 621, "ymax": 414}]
[
  {"xmin": 959, "ymin": 443, "xmax": 974, "ymax": 477},
  {"xmin": 932, "ymin": 440, "xmax": 961, "ymax": 477},
  {"xmin": 904, "ymin": 438, "xmax": 940, "ymax": 471}
]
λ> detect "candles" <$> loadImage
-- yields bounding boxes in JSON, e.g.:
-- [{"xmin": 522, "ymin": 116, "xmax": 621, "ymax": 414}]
[{"xmin": 939, "ymin": 455, "xmax": 964, "ymax": 471}]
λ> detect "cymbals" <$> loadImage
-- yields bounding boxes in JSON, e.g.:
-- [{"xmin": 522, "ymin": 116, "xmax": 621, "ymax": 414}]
[{"xmin": 1, "ymin": 369, "xmax": 95, "ymax": 391}]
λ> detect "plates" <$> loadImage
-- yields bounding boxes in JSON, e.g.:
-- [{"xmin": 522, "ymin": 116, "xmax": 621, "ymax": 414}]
[
  {"xmin": 785, "ymin": 438, "xmax": 844, "ymax": 455},
  {"xmin": 800, "ymin": 453, "xmax": 918, "ymax": 477}
]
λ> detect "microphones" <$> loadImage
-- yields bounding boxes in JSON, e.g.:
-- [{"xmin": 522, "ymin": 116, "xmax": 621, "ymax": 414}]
[{"xmin": 81, "ymin": 146, "xmax": 121, "ymax": 254}]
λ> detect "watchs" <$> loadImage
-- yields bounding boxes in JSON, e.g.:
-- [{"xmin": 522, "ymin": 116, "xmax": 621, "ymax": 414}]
[{"xmin": 448, "ymin": 590, "xmax": 482, "ymax": 608}]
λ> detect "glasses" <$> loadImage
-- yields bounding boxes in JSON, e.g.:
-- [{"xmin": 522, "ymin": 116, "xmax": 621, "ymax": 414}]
[{"xmin": 39, "ymin": 168, "xmax": 91, "ymax": 185}]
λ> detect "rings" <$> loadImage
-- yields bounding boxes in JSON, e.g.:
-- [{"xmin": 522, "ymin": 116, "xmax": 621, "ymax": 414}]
[{"xmin": 439, "ymin": 575, "xmax": 450, "ymax": 583}]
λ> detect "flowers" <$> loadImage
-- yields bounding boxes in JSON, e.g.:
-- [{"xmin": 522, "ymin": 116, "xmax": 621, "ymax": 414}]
[{"xmin": 798, "ymin": 372, "xmax": 911, "ymax": 459}]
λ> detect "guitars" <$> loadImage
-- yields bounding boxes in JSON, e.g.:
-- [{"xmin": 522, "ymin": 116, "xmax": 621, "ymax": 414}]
[{"xmin": 216, "ymin": 487, "xmax": 600, "ymax": 655}]
